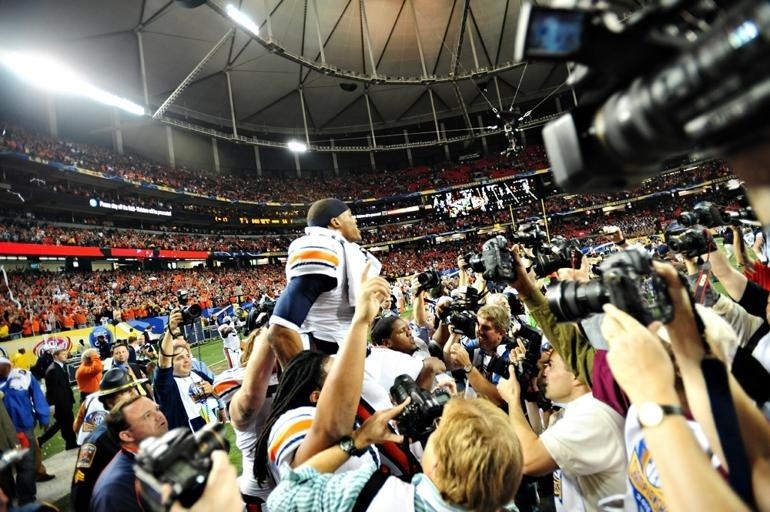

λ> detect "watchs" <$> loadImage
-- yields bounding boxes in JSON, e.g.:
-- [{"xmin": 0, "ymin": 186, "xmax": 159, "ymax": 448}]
[
  {"xmin": 338, "ymin": 436, "xmax": 371, "ymax": 459},
  {"xmin": 635, "ymin": 403, "xmax": 687, "ymax": 431}
]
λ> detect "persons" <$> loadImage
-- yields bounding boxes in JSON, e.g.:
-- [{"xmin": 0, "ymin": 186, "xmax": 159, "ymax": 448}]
[
  {"xmin": 76, "ymin": 340, "xmax": 89, "ymax": 356},
  {"xmin": 76, "ymin": 347, "xmax": 101, "ymax": 401},
  {"xmin": 670, "ymin": 180, "xmax": 760, "ymax": 210},
  {"xmin": 0, "ymin": 262, "xmax": 283, "ymax": 335},
  {"xmin": 2, "ymin": 360, "xmax": 56, "ymax": 481},
  {"xmin": 444, "ymin": 308, "xmax": 556, "ymax": 512},
  {"xmin": 148, "ymin": 308, "xmax": 228, "ymax": 443},
  {"xmin": 38, "ymin": 350, "xmax": 78, "ymax": 451},
  {"xmin": 499, "ymin": 344, "xmax": 634, "ymax": 512},
  {"xmin": 360, "ymin": 317, "xmax": 455, "ymax": 408},
  {"xmin": 8, "ymin": 349, "xmax": 34, "ymax": 379},
  {"xmin": 719, "ymin": 226, "xmax": 734, "ymax": 258},
  {"xmin": 0, "ymin": 119, "xmax": 551, "ymax": 205},
  {"xmin": 252, "ymin": 280, "xmax": 384, "ymax": 483},
  {"xmin": 90, "ymin": 399, "xmax": 167, "ymax": 511},
  {"xmin": 268, "ymin": 199, "xmax": 382, "ymax": 369},
  {"xmin": 547, "ymin": 188, "xmax": 671, "ymax": 237},
  {"xmin": 682, "ymin": 258, "xmax": 770, "ymax": 412},
  {"xmin": 699, "ymin": 228, "xmax": 770, "ymax": 321},
  {"xmin": 134, "ymin": 334, "xmax": 157, "ymax": 366},
  {"xmin": 599, "ymin": 259, "xmax": 770, "ymax": 511},
  {"xmin": 127, "ymin": 329, "xmax": 136, "ymax": 341},
  {"xmin": 96, "ymin": 336, "xmax": 110, "ymax": 360},
  {"xmin": 744, "ymin": 228, "xmax": 770, "ymax": 263},
  {"xmin": 643, "ymin": 158, "xmax": 737, "ymax": 190},
  {"xmin": 2, "ymin": 219, "xmax": 313, "ymax": 254},
  {"xmin": 646, "ymin": 244, "xmax": 656, "ymax": 259},
  {"xmin": 126, "ymin": 337, "xmax": 139, "ymax": 378},
  {"xmin": 109, "ymin": 346, "xmax": 143, "ymax": 391},
  {"xmin": 218, "ymin": 316, "xmax": 243, "ymax": 369},
  {"xmin": 599, "ymin": 252, "xmax": 609, "ymax": 262},
  {"xmin": 651, "ymin": 239, "xmax": 665, "ymax": 254},
  {"xmin": 211, "ymin": 306, "xmax": 278, "ymax": 512},
  {"xmin": 141, "ymin": 326, "xmax": 153, "ymax": 350},
  {"xmin": 357, "ymin": 206, "xmax": 554, "ymax": 311},
  {"xmin": 71, "ymin": 370, "xmax": 151, "ymax": 511},
  {"xmin": 265, "ymin": 395, "xmax": 524, "ymax": 511}
]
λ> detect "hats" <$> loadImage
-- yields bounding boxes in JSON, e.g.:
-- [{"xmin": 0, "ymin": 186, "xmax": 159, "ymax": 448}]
[
  {"xmin": 83, "ymin": 367, "xmax": 150, "ymax": 400},
  {"xmin": 304, "ymin": 197, "xmax": 350, "ymax": 229}
]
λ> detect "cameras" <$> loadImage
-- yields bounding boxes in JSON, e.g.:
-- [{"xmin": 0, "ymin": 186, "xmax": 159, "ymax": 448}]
[
  {"xmin": 511, "ymin": 225, "xmax": 546, "ymax": 245},
  {"xmin": 525, "ymin": 240, "xmax": 583, "ymax": 280},
  {"xmin": 720, "ymin": 226, "xmax": 742, "ymax": 246},
  {"xmin": 468, "ymin": 235, "xmax": 517, "ymax": 282},
  {"xmin": 515, "ymin": 0, "xmax": 769, "ymax": 196},
  {"xmin": 132, "ymin": 418, "xmax": 233, "ymax": 512},
  {"xmin": 663, "ymin": 221, "xmax": 718, "ymax": 258},
  {"xmin": 485, "ymin": 321, "xmax": 544, "ymax": 386},
  {"xmin": 389, "ymin": 373, "xmax": 452, "ymax": 442},
  {"xmin": 676, "ymin": 200, "xmax": 730, "ymax": 229},
  {"xmin": 461, "ymin": 250, "xmax": 480, "ymax": 270},
  {"xmin": 414, "ymin": 266, "xmax": 444, "ymax": 295},
  {"xmin": 173, "ymin": 302, "xmax": 203, "ymax": 326},
  {"xmin": 544, "ymin": 247, "xmax": 675, "ymax": 330},
  {"xmin": 445, "ymin": 308, "xmax": 478, "ymax": 340}
]
